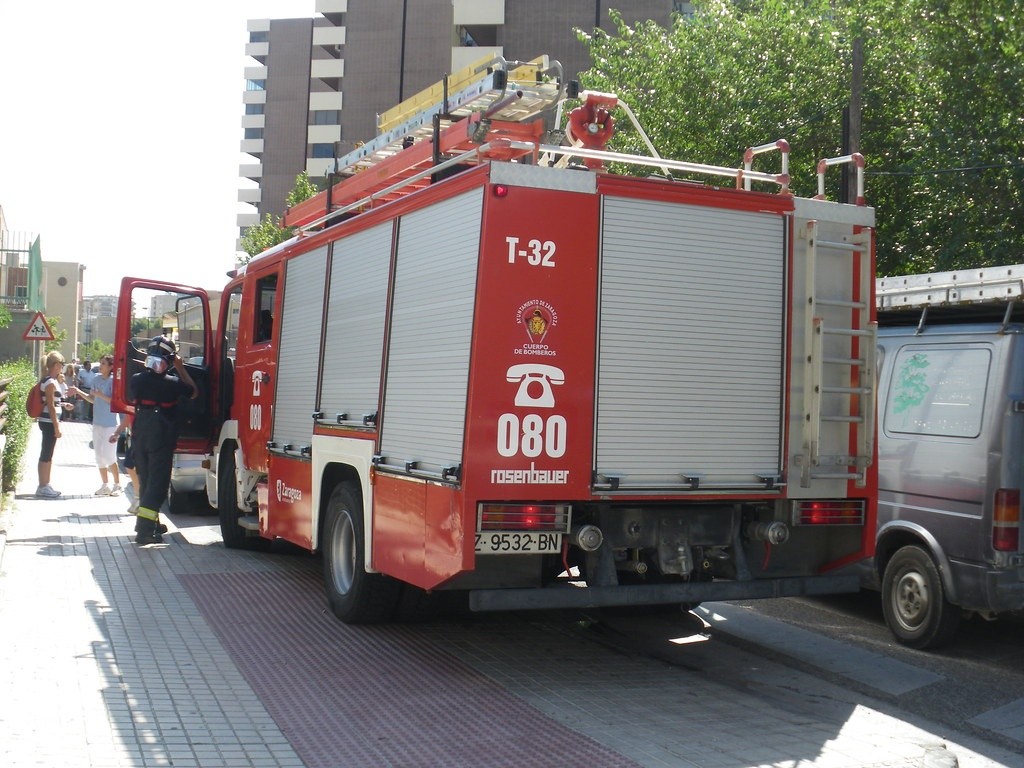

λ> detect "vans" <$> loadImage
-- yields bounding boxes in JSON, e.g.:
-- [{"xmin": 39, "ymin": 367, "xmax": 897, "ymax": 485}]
[{"xmin": 875, "ymin": 262, "xmax": 1024, "ymax": 652}]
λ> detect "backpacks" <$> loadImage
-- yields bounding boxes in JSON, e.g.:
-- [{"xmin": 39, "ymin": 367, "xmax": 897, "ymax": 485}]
[{"xmin": 26, "ymin": 376, "xmax": 50, "ymax": 418}]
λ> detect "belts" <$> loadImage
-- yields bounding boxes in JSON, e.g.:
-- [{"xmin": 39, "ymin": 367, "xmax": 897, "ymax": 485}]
[{"xmin": 83, "ymin": 387, "xmax": 92, "ymax": 390}]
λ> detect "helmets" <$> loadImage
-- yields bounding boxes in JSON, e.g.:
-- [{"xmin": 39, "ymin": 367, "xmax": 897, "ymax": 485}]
[{"xmin": 147, "ymin": 335, "xmax": 176, "ymax": 365}]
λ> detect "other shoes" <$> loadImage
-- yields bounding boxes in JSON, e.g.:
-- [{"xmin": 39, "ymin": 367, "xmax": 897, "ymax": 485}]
[
  {"xmin": 135, "ymin": 532, "xmax": 162, "ymax": 544},
  {"xmin": 127, "ymin": 496, "xmax": 140, "ymax": 513},
  {"xmin": 159, "ymin": 523, "xmax": 167, "ymax": 534}
]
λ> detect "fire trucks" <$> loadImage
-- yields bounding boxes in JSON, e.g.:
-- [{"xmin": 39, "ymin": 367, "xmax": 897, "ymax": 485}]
[{"xmin": 109, "ymin": 50, "xmax": 880, "ymax": 627}]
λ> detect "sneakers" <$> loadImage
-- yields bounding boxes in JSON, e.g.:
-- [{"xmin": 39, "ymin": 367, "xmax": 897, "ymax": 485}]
[
  {"xmin": 35, "ymin": 485, "xmax": 61, "ymax": 497},
  {"xmin": 95, "ymin": 485, "xmax": 112, "ymax": 495},
  {"xmin": 109, "ymin": 485, "xmax": 124, "ymax": 496}
]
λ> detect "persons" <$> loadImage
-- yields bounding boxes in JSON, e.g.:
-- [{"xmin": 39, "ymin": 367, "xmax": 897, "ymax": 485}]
[
  {"xmin": 65, "ymin": 354, "xmax": 125, "ymax": 496},
  {"xmin": 109, "ymin": 411, "xmax": 141, "ymax": 513},
  {"xmin": 125, "ymin": 334, "xmax": 200, "ymax": 545},
  {"xmin": 34, "ymin": 350, "xmax": 75, "ymax": 498},
  {"xmin": 56, "ymin": 359, "xmax": 100, "ymax": 424}
]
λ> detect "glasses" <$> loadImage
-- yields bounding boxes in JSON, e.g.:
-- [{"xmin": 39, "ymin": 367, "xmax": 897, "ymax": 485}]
[{"xmin": 60, "ymin": 362, "xmax": 66, "ymax": 366}]
